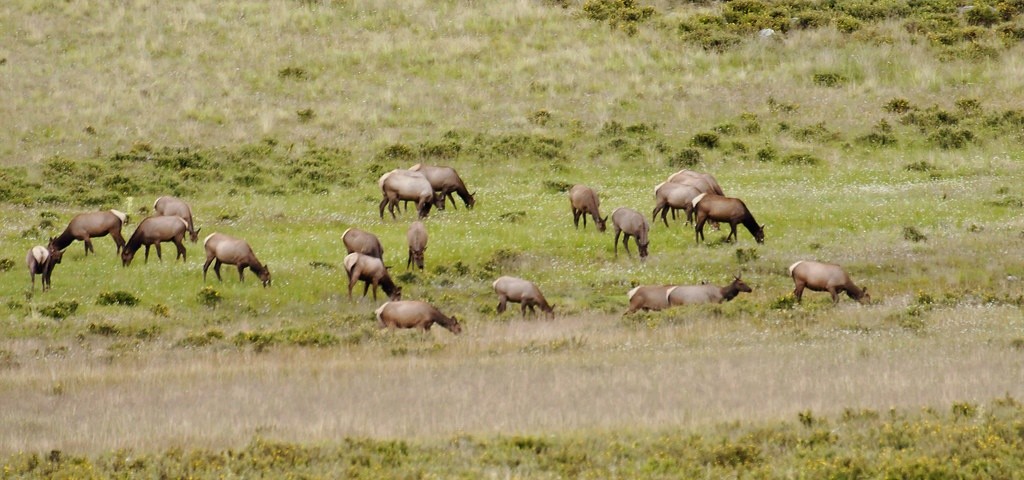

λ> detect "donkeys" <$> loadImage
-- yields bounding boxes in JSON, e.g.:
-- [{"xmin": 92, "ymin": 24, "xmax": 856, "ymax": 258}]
[{"xmin": 26, "ymin": 164, "xmax": 872, "ymax": 339}]
